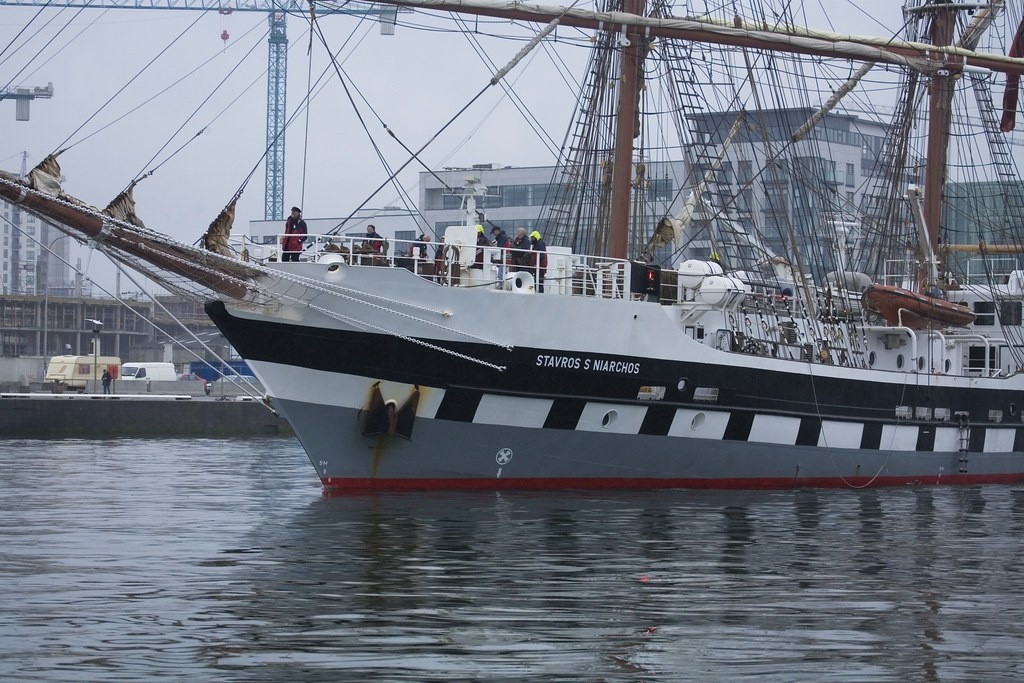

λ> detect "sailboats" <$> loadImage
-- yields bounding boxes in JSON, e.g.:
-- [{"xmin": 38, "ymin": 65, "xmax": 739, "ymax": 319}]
[{"xmin": 0, "ymin": 0, "xmax": 1024, "ymax": 500}]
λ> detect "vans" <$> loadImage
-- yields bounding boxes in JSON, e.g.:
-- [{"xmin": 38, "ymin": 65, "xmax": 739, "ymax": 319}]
[{"xmin": 122, "ymin": 362, "xmax": 176, "ymax": 382}]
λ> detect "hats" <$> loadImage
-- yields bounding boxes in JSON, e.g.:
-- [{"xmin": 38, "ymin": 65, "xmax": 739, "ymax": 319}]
[
  {"xmin": 490, "ymin": 226, "xmax": 500, "ymax": 235},
  {"xmin": 291, "ymin": 207, "xmax": 302, "ymax": 213},
  {"xmin": 709, "ymin": 252, "xmax": 719, "ymax": 262},
  {"xmin": 475, "ymin": 225, "xmax": 484, "ymax": 233},
  {"xmin": 529, "ymin": 231, "xmax": 541, "ymax": 242}
]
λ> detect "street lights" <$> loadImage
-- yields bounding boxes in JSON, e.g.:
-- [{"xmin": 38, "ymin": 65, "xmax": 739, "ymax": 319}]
[{"xmin": 85, "ymin": 319, "xmax": 103, "ymax": 394}]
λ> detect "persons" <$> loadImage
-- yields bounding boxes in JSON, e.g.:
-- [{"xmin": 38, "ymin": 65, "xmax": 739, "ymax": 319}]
[
  {"xmin": 282, "ymin": 207, "xmax": 308, "ymax": 262},
  {"xmin": 470, "ymin": 225, "xmax": 548, "ymax": 293},
  {"xmin": 361, "ymin": 225, "xmax": 384, "ymax": 253},
  {"xmin": 709, "ymin": 251, "xmax": 727, "ymax": 275},
  {"xmin": 101, "ymin": 369, "xmax": 111, "ymax": 394},
  {"xmin": 409, "ymin": 234, "xmax": 445, "ymax": 283}
]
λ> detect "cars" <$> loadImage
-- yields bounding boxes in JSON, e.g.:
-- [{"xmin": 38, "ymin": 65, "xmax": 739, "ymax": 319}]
[
  {"xmin": 206, "ymin": 375, "xmax": 260, "ymax": 390},
  {"xmin": 177, "ymin": 372, "xmax": 207, "ymax": 390}
]
